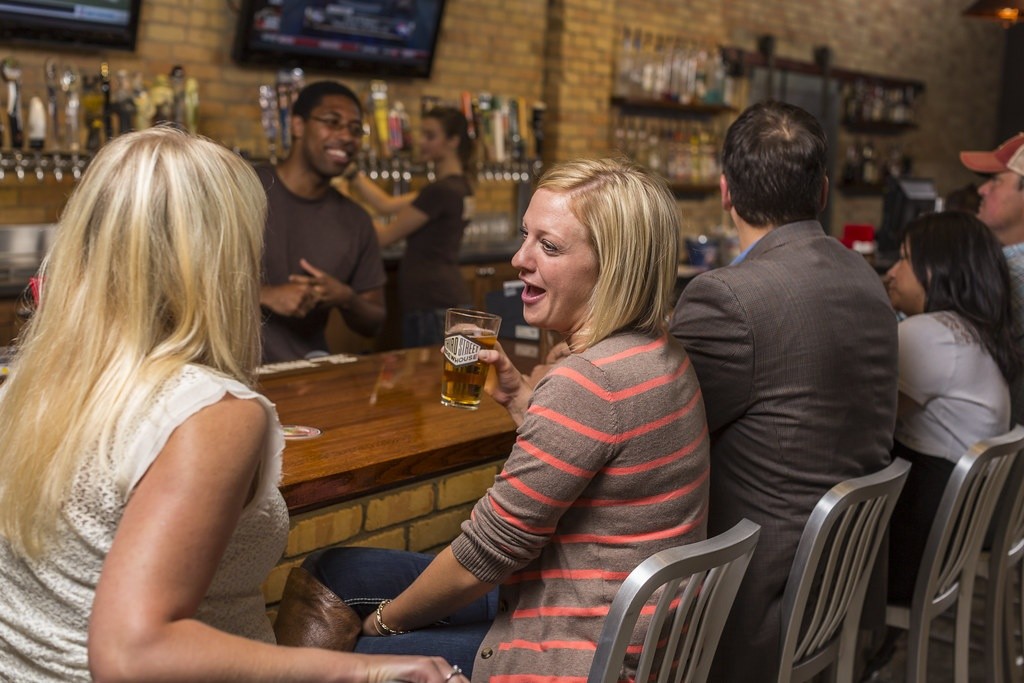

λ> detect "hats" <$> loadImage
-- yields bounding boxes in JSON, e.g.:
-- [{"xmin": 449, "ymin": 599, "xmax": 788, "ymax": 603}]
[{"xmin": 960, "ymin": 132, "xmax": 1024, "ymax": 173}]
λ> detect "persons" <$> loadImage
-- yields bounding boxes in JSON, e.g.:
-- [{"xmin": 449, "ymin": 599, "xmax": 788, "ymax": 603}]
[
  {"xmin": 251, "ymin": 80, "xmax": 385, "ymax": 364},
  {"xmin": 958, "ymin": 133, "xmax": 1024, "ymax": 351},
  {"xmin": 327, "ymin": 105, "xmax": 471, "ymax": 350},
  {"xmin": 521, "ymin": 98, "xmax": 898, "ymax": 683},
  {"xmin": 272, "ymin": 160, "xmax": 711, "ymax": 683},
  {"xmin": 885, "ymin": 208, "xmax": 1024, "ymax": 605},
  {"xmin": 0, "ymin": 125, "xmax": 472, "ymax": 683}
]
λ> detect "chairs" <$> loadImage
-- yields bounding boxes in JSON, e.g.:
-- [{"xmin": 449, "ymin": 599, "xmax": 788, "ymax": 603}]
[
  {"xmin": 756, "ymin": 457, "xmax": 911, "ymax": 683},
  {"xmin": 884, "ymin": 424, "xmax": 1024, "ymax": 683},
  {"xmin": 587, "ymin": 517, "xmax": 762, "ymax": 683}
]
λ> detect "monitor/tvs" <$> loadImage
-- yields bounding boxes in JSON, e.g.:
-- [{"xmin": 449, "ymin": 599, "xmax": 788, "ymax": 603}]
[
  {"xmin": 0, "ymin": 0, "xmax": 142, "ymax": 52},
  {"xmin": 229, "ymin": 0, "xmax": 447, "ymax": 81}
]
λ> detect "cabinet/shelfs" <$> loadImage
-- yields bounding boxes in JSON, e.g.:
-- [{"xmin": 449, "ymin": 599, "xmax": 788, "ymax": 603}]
[{"xmin": 610, "ymin": 49, "xmax": 925, "ymax": 198}]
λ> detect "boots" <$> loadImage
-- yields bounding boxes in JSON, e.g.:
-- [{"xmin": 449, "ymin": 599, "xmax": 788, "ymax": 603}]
[{"xmin": 273, "ymin": 567, "xmax": 361, "ymax": 652}]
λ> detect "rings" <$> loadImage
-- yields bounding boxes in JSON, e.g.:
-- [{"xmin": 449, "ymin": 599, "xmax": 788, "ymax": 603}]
[{"xmin": 445, "ymin": 664, "xmax": 462, "ymax": 681}]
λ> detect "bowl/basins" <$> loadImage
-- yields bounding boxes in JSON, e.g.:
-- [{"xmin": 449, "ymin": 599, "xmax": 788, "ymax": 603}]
[{"xmin": 1, "ymin": 224, "xmax": 59, "ymax": 271}]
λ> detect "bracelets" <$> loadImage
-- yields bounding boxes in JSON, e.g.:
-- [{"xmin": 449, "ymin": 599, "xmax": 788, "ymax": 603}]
[
  {"xmin": 346, "ymin": 167, "xmax": 360, "ymax": 179},
  {"xmin": 373, "ymin": 598, "xmax": 409, "ymax": 637}
]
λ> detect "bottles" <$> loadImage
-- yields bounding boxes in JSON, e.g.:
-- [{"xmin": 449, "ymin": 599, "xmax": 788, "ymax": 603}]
[
  {"xmin": 618, "ymin": 24, "xmax": 733, "ymax": 106},
  {"xmin": 609, "ymin": 112, "xmax": 723, "ymax": 187}
]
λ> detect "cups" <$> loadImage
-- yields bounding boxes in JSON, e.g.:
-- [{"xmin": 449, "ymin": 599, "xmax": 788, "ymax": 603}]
[{"xmin": 440, "ymin": 307, "xmax": 502, "ymax": 409}]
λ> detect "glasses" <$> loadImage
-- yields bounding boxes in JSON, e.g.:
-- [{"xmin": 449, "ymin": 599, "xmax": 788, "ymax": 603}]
[{"xmin": 304, "ymin": 114, "xmax": 364, "ymax": 137}]
